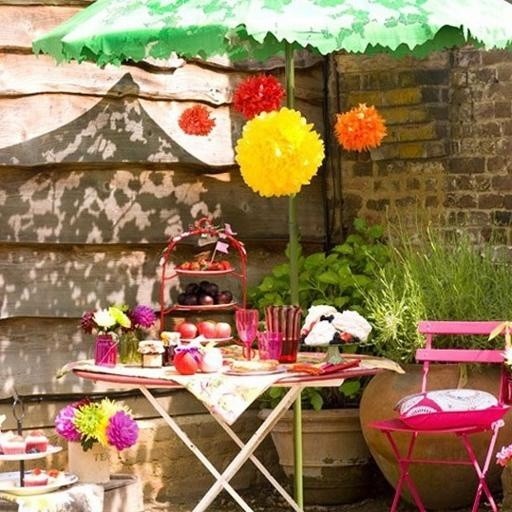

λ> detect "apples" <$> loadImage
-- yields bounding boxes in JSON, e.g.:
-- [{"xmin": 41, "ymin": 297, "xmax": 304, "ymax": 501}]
[
  {"xmin": 175, "ymin": 351, "xmax": 197, "ymax": 374},
  {"xmin": 178, "ymin": 320, "xmax": 231, "ymax": 339},
  {"xmin": 202, "ymin": 350, "xmax": 222, "ymax": 372}
]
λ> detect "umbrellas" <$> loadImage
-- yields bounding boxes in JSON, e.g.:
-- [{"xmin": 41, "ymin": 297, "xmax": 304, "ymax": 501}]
[{"xmin": 28, "ymin": 0, "xmax": 512, "ymax": 511}]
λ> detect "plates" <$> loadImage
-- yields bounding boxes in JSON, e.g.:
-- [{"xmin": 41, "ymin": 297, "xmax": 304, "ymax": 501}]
[
  {"xmin": 179, "ymin": 336, "xmax": 234, "ymax": 345},
  {"xmin": 173, "ymin": 299, "xmax": 237, "ymax": 312},
  {"xmin": 223, "ymin": 365, "xmax": 287, "ymax": 376},
  {"xmin": 174, "ymin": 267, "xmax": 235, "ymax": 277}
]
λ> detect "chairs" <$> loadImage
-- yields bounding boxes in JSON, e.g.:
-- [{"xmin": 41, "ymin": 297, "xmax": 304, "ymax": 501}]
[{"xmin": 363, "ymin": 320, "xmax": 512, "ymax": 511}]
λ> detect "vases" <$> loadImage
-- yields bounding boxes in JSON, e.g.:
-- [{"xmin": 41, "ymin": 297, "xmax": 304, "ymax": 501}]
[
  {"xmin": 93, "ymin": 330, "xmax": 141, "ymax": 366},
  {"xmin": 66, "ymin": 442, "xmax": 111, "ymax": 485}
]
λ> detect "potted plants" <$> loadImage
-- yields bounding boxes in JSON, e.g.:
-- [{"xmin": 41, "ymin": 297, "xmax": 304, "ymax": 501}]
[
  {"xmin": 248, "ymin": 214, "xmax": 402, "ymax": 507},
  {"xmin": 359, "ymin": 188, "xmax": 512, "ymax": 510}
]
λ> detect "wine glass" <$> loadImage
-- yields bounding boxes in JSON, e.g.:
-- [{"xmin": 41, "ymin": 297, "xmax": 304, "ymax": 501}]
[{"xmin": 236, "ymin": 309, "xmax": 260, "ymax": 360}]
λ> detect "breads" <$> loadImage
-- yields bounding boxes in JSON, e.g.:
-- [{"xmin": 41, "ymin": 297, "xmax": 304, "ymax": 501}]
[{"xmin": 230, "ymin": 360, "xmax": 280, "ymax": 372}]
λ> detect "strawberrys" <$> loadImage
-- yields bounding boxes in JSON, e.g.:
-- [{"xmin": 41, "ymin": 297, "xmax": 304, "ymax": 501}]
[{"xmin": 181, "ymin": 259, "xmax": 229, "ymax": 271}]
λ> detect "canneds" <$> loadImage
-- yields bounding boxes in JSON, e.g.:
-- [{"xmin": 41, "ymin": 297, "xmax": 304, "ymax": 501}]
[
  {"xmin": 137, "ymin": 340, "xmax": 165, "ymax": 368},
  {"xmin": 160, "ymin": 331, "xmax": 181, "ymax": 366}
]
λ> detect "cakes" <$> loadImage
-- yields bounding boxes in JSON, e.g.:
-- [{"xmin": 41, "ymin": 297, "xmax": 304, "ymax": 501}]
[
  {"xmin": 302, "ymin": 305, "xmax": 372, "ymax": 345},
  {"xmin": 0, "ymin": 430, "xmax": 48, "ymax": 454},
  {"xmin": 22, "ymin": 467, "xmax": 64, "ymax": 487}
]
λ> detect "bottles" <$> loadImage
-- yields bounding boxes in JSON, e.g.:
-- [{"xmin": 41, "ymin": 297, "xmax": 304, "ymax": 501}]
[
  {"xmin": 120, "ymin": 330, "xmax": 143, "ymax": 365},
  {"xmin": 95, "ymin": 333, "xmax": 117, "ymax": 366}
]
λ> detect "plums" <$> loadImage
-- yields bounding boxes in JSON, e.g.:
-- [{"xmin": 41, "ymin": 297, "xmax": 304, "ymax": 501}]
[{"xmin": 178, "ymin": 280, "xmax": 233, "ymax": 304}]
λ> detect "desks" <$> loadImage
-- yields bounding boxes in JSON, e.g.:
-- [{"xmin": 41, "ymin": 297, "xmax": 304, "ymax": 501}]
[
  {"xmin": 56, "ymin": 352, "xmax": 405, "ymax": 512},
  {"xmin": 0, "ymin": 473, "xmax": 143, "ymax": 512}
]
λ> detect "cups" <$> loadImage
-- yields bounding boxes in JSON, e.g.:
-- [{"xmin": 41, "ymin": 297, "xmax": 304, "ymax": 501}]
[
  {"xmin": 256, "ymin": 331, "xmax": 282, "ymax": 360},
  {"xmin": 265, "ymin": 304, "xmax": 303, "ymax": 364}
]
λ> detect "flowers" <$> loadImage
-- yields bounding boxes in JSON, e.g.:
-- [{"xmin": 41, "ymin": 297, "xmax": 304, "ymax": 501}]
[
  {"xmin": 55, "ymin": 398, "xmax": 137, "ymax": 449},
  {"xmin": 80, "ymin": 303, "xmax": 155, "ymax": 358}
]
